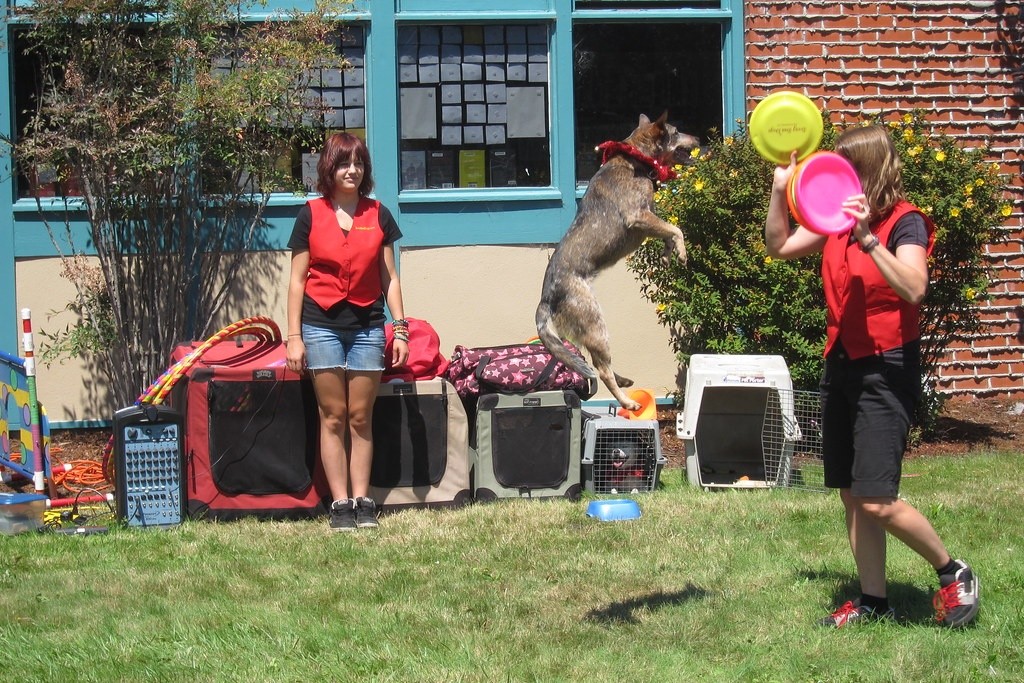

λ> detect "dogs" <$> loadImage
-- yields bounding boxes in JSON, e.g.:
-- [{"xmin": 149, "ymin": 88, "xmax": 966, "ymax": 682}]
[
  {"xmin": 534, "ymin": 108, "xmax": 701, "ymax": 413},
  {"xmin": 602, "ymin": 440, "xmax": 648, "ymax": 495}
]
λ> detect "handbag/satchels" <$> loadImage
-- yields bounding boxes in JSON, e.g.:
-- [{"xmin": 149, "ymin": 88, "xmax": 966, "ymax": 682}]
[{"xmin": 443, "ymin": 339, "xmax": 589, "ymax": 408}]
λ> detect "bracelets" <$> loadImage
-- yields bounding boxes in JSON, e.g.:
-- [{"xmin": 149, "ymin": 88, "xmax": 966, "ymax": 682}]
[
  {"xmin": 863, "ymin": 236, "xmax": 879, "ymax": 253},
  {"xmin": 287, "ymin": 334, "xmax": 301, "ymax": 337},
  {"xmin": 392, "ymin": 320, "xmax": 409, "ymax": 342}
]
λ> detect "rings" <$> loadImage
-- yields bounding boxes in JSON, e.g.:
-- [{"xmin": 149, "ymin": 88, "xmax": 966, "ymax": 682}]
[{"xmin": 861, "ymin": 206, "xmax": 866, "ymax": 212}]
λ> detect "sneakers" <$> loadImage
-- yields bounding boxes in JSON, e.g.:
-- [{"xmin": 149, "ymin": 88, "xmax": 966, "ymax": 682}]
[
  {"xmin": 355, "ymin": 496, "xmax": 379, "ymax": 528},
  {"xmin": 932, "ymin": 557, "xmax": 980, "ymax": 628},
  {"xmin": 329, "ymin": 498, "xmax": 358, "ymax": 532},
  {"xmin": 811, "ymin": 596, "xmax": 896, "ymax": 630}
]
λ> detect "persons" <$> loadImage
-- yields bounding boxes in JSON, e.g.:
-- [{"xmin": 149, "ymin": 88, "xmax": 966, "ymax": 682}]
[
  {"xmin": 286, "ymin": 133, "xmax": 410, "ymax": 533},
  {"xmin": 766, "ymin": 127, "xmax": 979, "ymax": 630}
]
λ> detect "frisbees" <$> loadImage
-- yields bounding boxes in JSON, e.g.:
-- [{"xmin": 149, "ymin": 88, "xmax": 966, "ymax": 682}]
[
  {"xmin": 786, "ymin": 150, "xmax": 864, "ymax": 236},
  {"xmin": 748, "ymin": 90, "xmax": 824, "ymax": 167}
]
